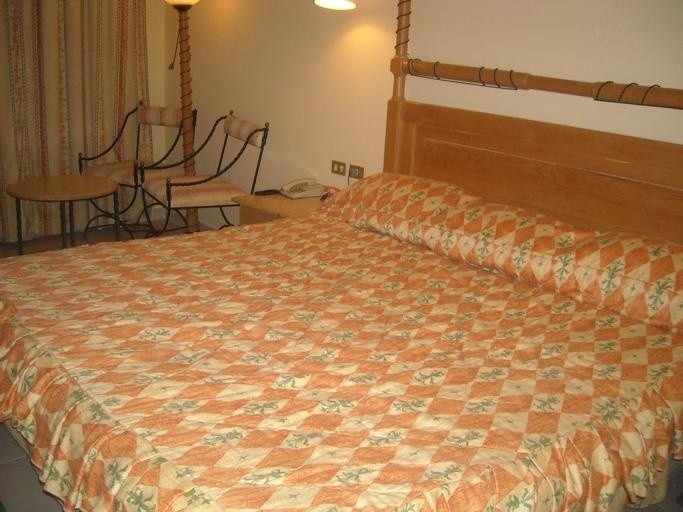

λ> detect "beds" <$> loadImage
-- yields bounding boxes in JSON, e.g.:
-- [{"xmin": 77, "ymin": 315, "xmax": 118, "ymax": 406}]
[{"xmin": 0, "ymin": 1, "xmax": 683, "ymax": 510}]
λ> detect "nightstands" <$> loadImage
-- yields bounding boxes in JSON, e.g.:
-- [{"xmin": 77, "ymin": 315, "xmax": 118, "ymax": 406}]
[{"xmin": 230, "ymin": 195, "xmax": 323, "ymax": 227}]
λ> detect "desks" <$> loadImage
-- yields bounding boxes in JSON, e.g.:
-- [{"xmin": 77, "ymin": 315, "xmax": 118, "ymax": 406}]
[{"xmin": 7, "ymin": 176, "xmax": 120, "ymax": 256}]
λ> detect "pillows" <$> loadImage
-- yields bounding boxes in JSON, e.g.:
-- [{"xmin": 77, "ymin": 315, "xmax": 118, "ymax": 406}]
[{"xmin": 317, "ymin": 172, "xmax": 683, "ymax": 333}]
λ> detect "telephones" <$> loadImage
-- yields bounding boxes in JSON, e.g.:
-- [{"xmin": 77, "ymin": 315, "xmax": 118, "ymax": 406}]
[{"xmin": 279, "ymin": 177, "xmax": 325, "ymax": 200}]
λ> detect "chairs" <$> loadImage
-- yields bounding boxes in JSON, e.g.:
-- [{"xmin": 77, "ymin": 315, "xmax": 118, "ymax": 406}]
[{"xmin": 78, "ymin": 100, "xmax": 269, "ymax": 243}]
